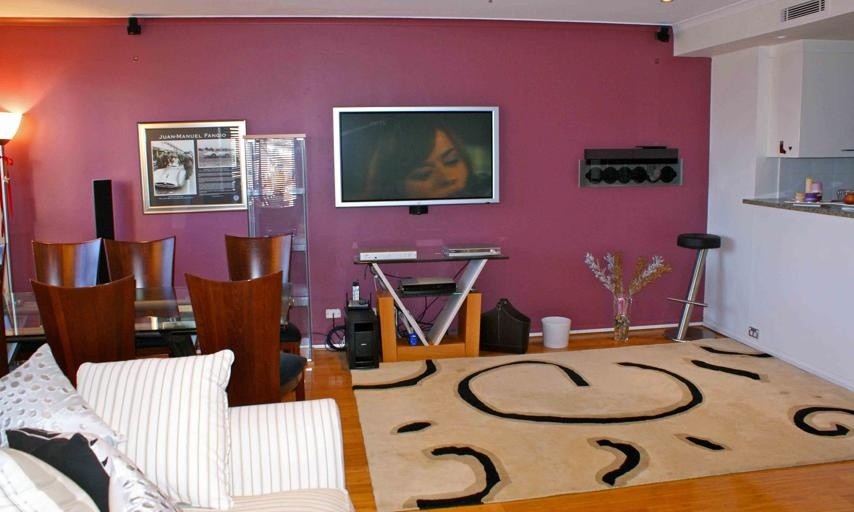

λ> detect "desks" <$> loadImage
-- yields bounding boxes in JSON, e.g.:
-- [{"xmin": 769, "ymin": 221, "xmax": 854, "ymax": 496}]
[{"xmin": 4, "ymin": 283, "xmax": 294, "ymax": 356}]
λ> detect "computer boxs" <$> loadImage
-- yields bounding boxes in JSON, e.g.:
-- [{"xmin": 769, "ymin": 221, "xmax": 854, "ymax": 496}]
[{"xmin": 344, "ymin": 310, "xmax": 380, "ymax": 369}]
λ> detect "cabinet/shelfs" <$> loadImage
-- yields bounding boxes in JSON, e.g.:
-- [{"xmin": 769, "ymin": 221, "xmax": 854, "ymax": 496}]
[
  {"xmin": 352, "ymin": 253, "xmax": 510, "ymax": 362},
  {"xmin": 244, "ymin": 134, "xmax": 314, "ymax": 361},
  {"xmin": 760, "ymin": 38, "xmax": 854, "ymax": 157}
]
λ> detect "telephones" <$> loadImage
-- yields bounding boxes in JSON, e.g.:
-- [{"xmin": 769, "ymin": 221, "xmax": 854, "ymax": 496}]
[{"xmin": 346, "ymin": 280, "xmax": 371, "ymax": 311}]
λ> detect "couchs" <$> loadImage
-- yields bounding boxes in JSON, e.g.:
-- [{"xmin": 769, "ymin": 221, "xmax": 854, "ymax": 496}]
[{"xmin": 0, "ymin": 398, "xmax": 355, "ymax": 512}]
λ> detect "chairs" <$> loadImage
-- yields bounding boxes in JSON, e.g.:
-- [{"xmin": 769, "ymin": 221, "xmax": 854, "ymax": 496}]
[
  {"xmin": 30, "ymin": 238, "xmax": 102, "ymax": 327},
  {"xmin": 30, "ymin": 274, "xmax": 136, "ymax": 389},
  {"xmin": 102, "ymin": 235, "xmax": 180, "ymax": 357},
  {"xmin": 224, "ymin": 232, "xmax": 301, "ymax": 354},
  {"xmin": 184, "ymin": 270, "xmax": 307, "ymax": 407}
]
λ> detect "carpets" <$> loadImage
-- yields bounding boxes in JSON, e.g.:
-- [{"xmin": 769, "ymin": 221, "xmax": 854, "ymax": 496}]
[{"xmin": 349, "ymin": 337, "xmax": 853, "ymax": 511}]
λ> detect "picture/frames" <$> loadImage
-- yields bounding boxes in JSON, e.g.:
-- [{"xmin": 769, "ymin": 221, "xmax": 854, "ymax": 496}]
[{"xmin": 137, "ymin": 120, "xmax": 248, "ymax": 214}]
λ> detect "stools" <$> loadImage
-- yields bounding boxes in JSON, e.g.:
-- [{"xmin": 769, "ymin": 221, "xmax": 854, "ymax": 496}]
[{"xmin": 664, "ymin": 232, "xmax": 720, "ymax": 342}]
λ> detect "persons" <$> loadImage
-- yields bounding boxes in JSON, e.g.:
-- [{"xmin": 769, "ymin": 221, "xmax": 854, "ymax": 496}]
[{"xmin": 360, "ymin": 117, "xmax": 474, "ymax": 199}]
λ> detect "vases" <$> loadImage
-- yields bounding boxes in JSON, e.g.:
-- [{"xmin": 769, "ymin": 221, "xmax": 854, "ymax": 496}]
[{"xmin": 611, "ymin": 295, "xmax": 632, "ymax": 343}]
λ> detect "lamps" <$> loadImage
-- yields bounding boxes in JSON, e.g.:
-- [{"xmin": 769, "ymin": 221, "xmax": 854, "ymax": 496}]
[{"xmin": 0, "ymin": 111, "xmax": 23, "ymax": 336}]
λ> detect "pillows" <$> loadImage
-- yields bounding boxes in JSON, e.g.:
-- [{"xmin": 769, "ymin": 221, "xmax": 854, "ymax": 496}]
[
  {"xmin": 5, "ymin": 428, "xmax": 108, "ymax": 512},
  {"xmin": 83, "ymin": 432, "xmax": 177, "ymax": 512},
  {"xmin": 77, "ymin": 349, "xmax": 234, "ymax": 512},
  {"xmin": 1, "ymin": 342, "xmax": 123, "ymax": 447}
]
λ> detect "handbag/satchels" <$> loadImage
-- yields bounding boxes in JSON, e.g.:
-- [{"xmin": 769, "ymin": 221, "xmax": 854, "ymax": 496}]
[{"xmin": 480, "ymin": 297, "xmax": 531, "ymax": 353}]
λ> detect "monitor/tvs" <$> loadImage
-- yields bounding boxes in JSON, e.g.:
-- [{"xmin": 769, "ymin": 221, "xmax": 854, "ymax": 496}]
[{"xmin": 333, "ymin": 105, "xmax": 500, "ymax": 208}]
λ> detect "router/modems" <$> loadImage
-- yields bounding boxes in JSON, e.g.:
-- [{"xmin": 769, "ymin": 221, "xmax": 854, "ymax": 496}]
[{"xmin": 345, "ymin": 292, "xmax": 372, "ymax": 310}]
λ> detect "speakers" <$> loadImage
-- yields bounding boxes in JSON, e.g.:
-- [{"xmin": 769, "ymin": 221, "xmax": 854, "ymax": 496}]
[{"xmin": 94, "ymin": 180, "xmax": 114, "ymax": 239}]
